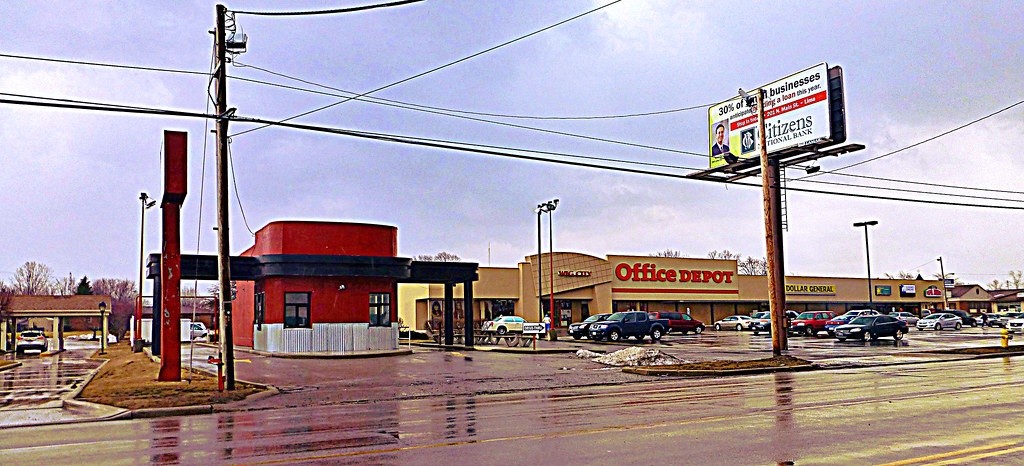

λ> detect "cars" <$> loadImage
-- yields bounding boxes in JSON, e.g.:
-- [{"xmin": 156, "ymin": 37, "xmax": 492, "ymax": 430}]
[
  {"xmin": 922, "ymin": 309, "xmax": 932, "ymax": 317},
  {"xmin": 939, "ymin": 309, "xmax": 978, "ymax": 327},
  {"xmin": 825, "ymin": 309, "xmax": 880, "ymax": 336},
  {"xmin": 713, "ymin": 314, "xmax": 753, "ymax": 331},
  {"xmin": 889, "ymin": 311, "xmax": 921, "ymax": 327},
  {"xmin": 748, "ymin": 310, "xmax": 799, "ymax": 336},
  {"xmin": 190, "ymin": 322, "xmax": 208, "ymax": 339},
  {"xmin": 790, "ymin": 311, "xmax": 842, "ymax": 336},
  {"xmin": 568, "ymin": 313, "xmax": 613, "ymax": 340},
  {"xmin": 833, "ymin": 314, "xmax": 910, "ymax": 342},
  {"xmin": 916, "ymin": 312, "xmax": 963, "ymax": 331},
  {"xmin": 16, "ymin": 329, "xmax": 48, "ymax": 353},
  {"xmin": 482, "ymin": 316, "xmax": 530, "ymax": 335},
  {"xmin": 972, "ymin": 311, "xmax": 1024, "ymax": 334}
]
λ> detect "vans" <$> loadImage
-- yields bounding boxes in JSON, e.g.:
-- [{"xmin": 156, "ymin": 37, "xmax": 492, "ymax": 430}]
[{"xmin": 649, "ymin": 311, "xmax": 707, "ymax": 335}]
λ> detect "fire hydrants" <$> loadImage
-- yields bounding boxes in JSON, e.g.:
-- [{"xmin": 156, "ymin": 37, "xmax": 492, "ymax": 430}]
[{"xmin": 1000, "ymin": 329, "xmax": 1014, "ymax": 348}]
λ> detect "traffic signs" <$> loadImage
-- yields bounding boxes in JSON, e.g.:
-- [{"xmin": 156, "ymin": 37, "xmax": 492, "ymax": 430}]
[{"xmin": 523, "ymin": 323, "xmax": 547, "ymax": 333}]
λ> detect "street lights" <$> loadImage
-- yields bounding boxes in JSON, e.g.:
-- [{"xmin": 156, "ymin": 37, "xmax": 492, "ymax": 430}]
[
  {"xmin": 544, "ymin": 198, "xmax": 560, "ymax": 329},
  {"xmin": 941, "ymin": 273, "xmax": 955, "ymax": 308},
  {"xmin": 98, "ymin": 301, "xmax": 107, "ymax": 354},
  {"xmin": 536, "ymin": 202, "xmax": 547, "ymax": 321},
  {"xmin": 852, "ymin": 220, "xmax": 879, "ymax": 310},
  {"xmin": 134, "ymin": 192, "xmax": 157, "ymax": 346}
]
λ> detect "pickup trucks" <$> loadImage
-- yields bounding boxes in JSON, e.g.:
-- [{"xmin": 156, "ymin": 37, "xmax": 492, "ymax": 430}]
[{"xmin": 588, "ymin": 310, "xmax": 671, "ymax": 342}]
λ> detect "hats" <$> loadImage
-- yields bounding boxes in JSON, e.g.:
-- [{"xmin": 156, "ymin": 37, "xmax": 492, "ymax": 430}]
[{"xmin": 545, "ymin": 313, "xmax": 549, "ymax": 317}]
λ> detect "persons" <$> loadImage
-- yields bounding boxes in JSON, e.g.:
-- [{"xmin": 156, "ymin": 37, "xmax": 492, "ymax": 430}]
[
  {"xmin": 712, "ymin": 123, "xmax": 730, "ymax": 156},
  {"xmin": 543, "ymin": 314, "xmax": 551, "ymax": 330},
  {"xmin": 431, "ymin": 301, "xmax": 491, "ymax": 328},
  {"xmin": 980, "ymin": 313, "xmax": 990, "ymax": 328}
]
